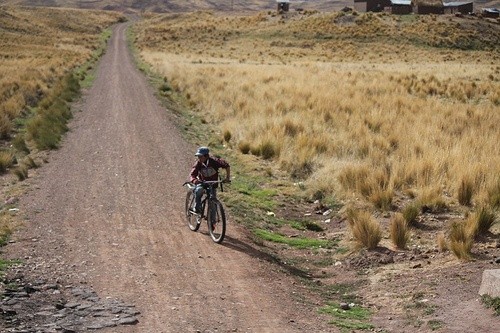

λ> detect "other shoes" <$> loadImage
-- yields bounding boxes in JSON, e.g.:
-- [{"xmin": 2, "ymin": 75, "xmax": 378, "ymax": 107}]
[{"xmin": 194, "ymin": 207, "xmax": 201, "ymax": 213}]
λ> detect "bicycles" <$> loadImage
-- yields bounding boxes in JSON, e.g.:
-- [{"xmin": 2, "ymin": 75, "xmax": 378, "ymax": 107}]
[{"xmin": 184, "ymin": 177, "xmax": 229, "ymax": 243}]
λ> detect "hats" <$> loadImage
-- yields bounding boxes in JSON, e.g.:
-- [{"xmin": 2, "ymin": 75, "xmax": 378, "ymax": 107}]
[{"xmin": 194, "ymin": 146, "xmax": 209, "ymax": 156}]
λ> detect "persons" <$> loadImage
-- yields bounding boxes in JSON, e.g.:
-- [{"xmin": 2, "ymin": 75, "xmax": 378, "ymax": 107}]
[{"xmin": 188, "ymin": 147, "xmax": 230, "ymax": 231}]
